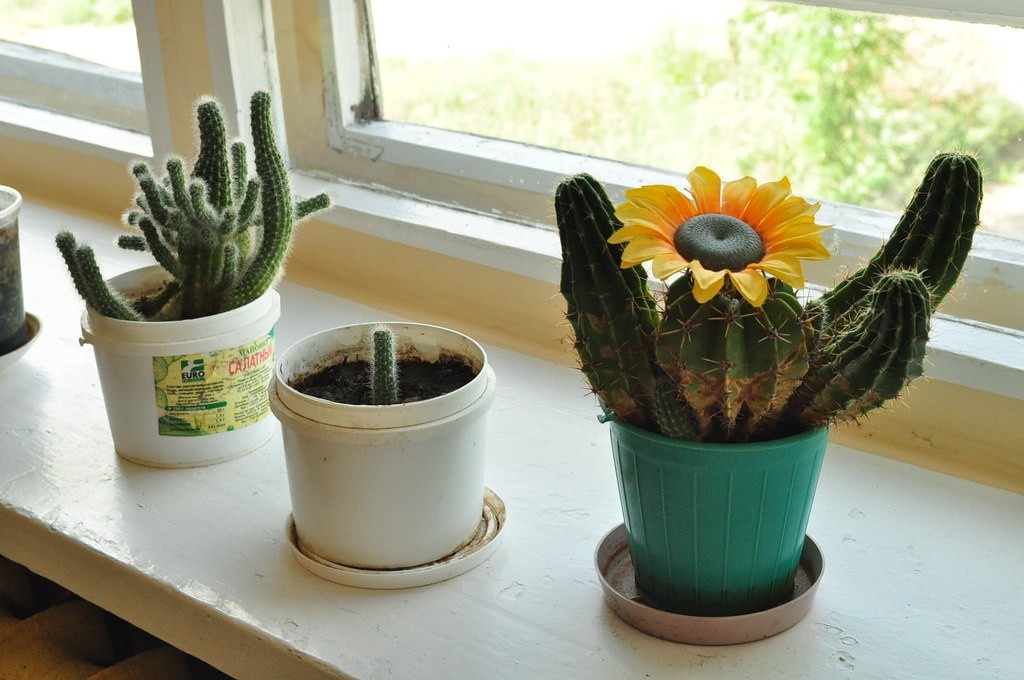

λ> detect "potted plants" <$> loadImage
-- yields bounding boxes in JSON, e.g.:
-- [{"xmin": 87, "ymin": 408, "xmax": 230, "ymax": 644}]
[
  {"xmin": 54, "ymin": 88, "xmax": 336, "ymax": 471},
  {"xmin": 267, "ymin": 322, "xmax": 497, "ymax": 571}
]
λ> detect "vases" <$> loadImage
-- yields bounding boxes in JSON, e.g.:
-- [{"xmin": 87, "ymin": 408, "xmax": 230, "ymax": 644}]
[
  {"xmin": 586, "ymin": 395, "xmax": 837, "ymax": 617},
  {"xmin": 0, "ymin": 184, "xmax": 43, "ymax": 374}
]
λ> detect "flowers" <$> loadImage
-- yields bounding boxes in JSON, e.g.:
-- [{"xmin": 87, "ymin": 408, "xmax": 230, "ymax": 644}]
[{"xmin": 546, "ymin": 144, "xmax": 992, "ymax": 447}]
